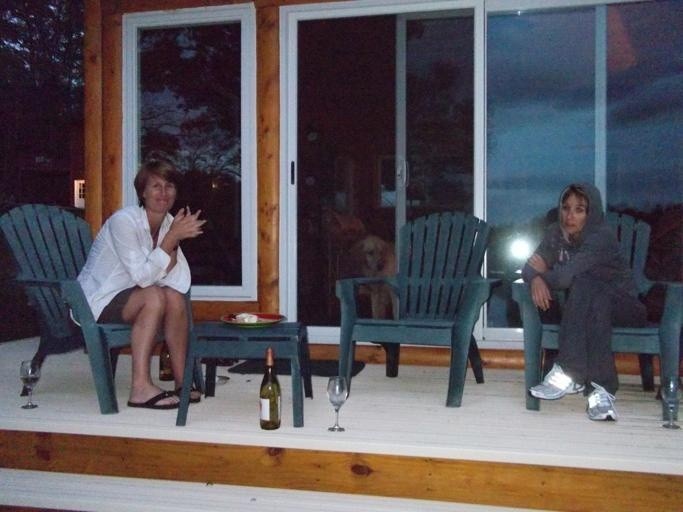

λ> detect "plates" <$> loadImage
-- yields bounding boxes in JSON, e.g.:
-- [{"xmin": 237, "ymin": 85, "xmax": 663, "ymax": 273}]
[{"xmin": 221, "ymin": 313, "xmax": 286, "ymax": 329}]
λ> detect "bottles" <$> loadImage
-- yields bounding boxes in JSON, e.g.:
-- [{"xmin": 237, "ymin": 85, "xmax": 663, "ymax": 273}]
[{"xmin": 258, "ymin": 346, "xmax": 283, "ymax": 432}]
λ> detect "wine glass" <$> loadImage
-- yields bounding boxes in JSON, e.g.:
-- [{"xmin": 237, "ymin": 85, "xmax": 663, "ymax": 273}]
[
  {"xmin": 657, "ymin": 374, "xmax": 680, "ymax": 430},
  {"xmin": 17, "ymin": 360, "xmax": 40, "ymax": 410},
  {"xmin": 325, "ymin": 377, "xmax": 347, "ymax": 434}
]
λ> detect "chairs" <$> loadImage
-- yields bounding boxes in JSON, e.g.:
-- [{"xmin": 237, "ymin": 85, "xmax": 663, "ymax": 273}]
[
  {"xmin": 0, "ymin": 203, "xmax": 203, "ymax": 421},
  {"xmin": 510, "ymin": 207, "xmax": 682, "ymax": 422},
  {"xmin": 329, "ymin": 211, "xmax": 502, "ymax": 410}
]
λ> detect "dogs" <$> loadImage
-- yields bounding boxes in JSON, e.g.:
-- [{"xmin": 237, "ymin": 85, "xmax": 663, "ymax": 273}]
[{"xmin": 355, "ymin": 235, "xmax": 395, "ymax": 320}]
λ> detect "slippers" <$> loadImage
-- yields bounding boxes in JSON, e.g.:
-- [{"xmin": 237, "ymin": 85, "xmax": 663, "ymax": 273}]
[
  {"xmin": 127, "ymin": 390, "xmax": 181, "ymax": 410},
  {"xmin": 174, "ymin": 385, "xmax": 202, "ymax": 403}
]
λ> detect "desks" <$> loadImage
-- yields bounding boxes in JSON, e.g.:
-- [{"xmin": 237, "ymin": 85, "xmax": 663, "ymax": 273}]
[{"xmin": 168, "ymin": 314, "xmax": 314, "ymax": 430}]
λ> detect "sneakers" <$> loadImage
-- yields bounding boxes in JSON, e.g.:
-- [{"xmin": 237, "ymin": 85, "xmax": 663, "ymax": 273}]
[
  {"xmin": 585, "ymin": 381, "xmax": 617, "ymax": 422},
  {"xmin": 527, "ymin": 360, "xmax": 586, "ymax": 401}
]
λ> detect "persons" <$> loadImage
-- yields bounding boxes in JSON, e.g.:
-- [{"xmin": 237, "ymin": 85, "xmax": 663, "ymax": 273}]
[
  {"xmin": 72, "ymin": 157, "xmax": 211, "ymax": 410},
  {"xmin": 521, "ymin": 182, "xmax": 650, "ymax": 421}
]
